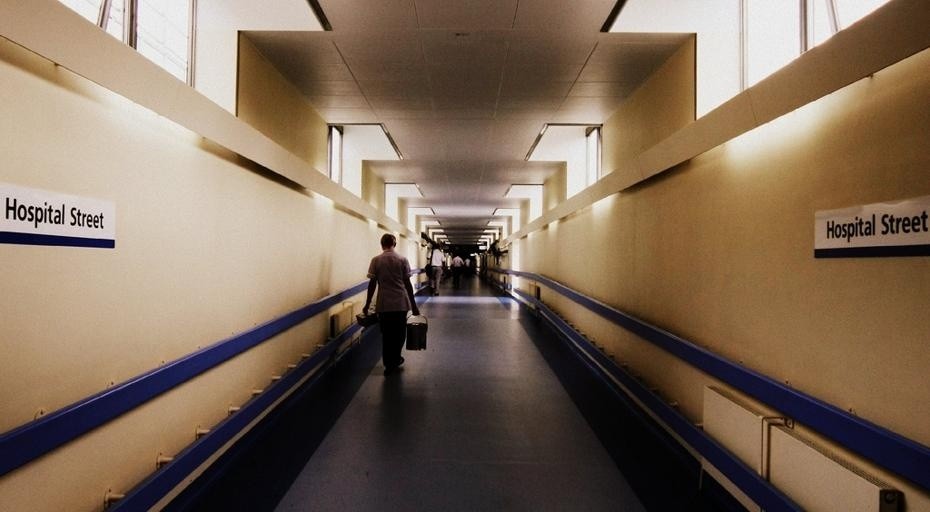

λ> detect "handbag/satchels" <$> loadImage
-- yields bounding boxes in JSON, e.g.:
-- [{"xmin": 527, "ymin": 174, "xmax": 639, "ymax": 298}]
[{"xmin": 425, "ymin": 264, "xmax": 432, "ymax": 277}]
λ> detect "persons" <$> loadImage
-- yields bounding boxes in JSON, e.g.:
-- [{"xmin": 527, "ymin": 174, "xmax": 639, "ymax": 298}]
[
  {"xmin": 363, "ymin": 233, "xmax": 420, "ymax": 376},
  {"xmin": 427, "ymin": 243, "xmax": 471, "ymax": 296}
]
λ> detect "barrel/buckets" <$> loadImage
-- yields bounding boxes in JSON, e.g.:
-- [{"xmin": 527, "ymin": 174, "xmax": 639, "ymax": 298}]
[{"xmin": 405, "ymin": 313, "xmax": 429, "ymax": 351}]
